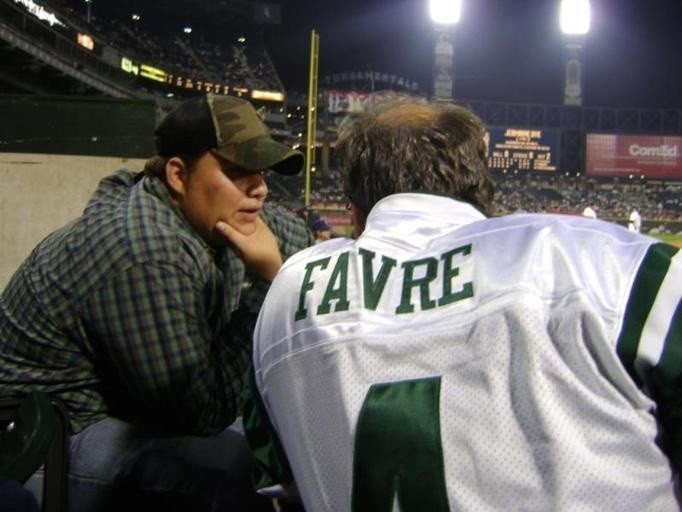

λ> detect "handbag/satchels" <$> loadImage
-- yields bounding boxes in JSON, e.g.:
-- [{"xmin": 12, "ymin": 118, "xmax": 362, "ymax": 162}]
[{"xmin": 1, "ymin": 393, "xmax": 62, "ymax": 483}]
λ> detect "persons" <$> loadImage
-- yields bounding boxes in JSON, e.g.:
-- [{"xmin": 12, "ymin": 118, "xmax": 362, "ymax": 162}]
[
  {"xmin": 236, "ymin": 85, "xmax": 682, "ymax": 512},
  {"xmin": 42, "ymin": 1, "xmax": 682, "ymax": 264},
  {"xmin": 0, "ymin": 91, "xmax": 315, "ymax": 511}
]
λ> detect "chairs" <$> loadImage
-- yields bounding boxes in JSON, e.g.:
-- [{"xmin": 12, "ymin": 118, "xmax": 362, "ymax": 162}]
[{"xmin": 0, "ymin": 390, "xmax": 71, "ymax": 512}]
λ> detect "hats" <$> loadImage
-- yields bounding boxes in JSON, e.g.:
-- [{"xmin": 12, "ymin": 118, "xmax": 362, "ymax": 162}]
[{"xmin": 155, "ymin": 92, "xmax": 304, "ymax": 176}]
